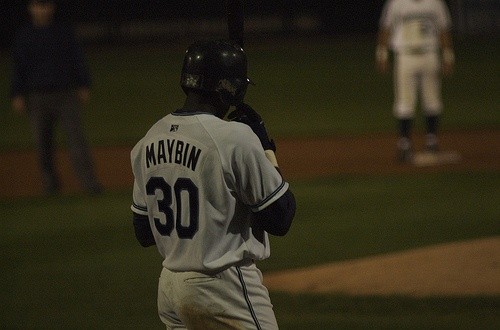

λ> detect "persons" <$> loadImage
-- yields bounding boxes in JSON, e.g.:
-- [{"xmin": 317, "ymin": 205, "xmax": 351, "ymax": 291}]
[
  {"xmin": 131, "ymin": 38, "xmax": 296, "ymax": 330},
  {"xmin": 377, "ymin": 0, "xmax": 457, "ymax": 155},
  {"xmin": 11, "ymin": 0, "xmax": 103, "ymax": 196}
]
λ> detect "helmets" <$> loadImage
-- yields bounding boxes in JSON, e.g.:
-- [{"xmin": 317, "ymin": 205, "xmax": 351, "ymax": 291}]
[{"xmin": 180, "ymin": 39, "xmax": 256, "ymax": 92}]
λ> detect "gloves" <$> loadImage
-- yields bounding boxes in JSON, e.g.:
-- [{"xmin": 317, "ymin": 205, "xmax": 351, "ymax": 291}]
[{"xmin": 228, "ymin": 103, "xmax": 276, "ymax": 153}]
